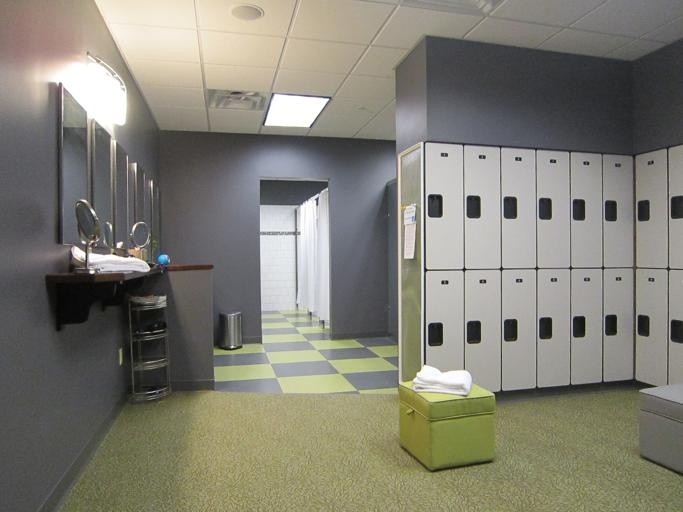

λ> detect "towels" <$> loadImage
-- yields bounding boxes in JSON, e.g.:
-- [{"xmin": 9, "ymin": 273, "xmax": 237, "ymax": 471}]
[
  {"xmin": 413, "ymin": 364, "xmax": 473, "ymax": 397},
  {"xmin": 69, "ymin": 242, "xmax": 152, "ymax": 274}
]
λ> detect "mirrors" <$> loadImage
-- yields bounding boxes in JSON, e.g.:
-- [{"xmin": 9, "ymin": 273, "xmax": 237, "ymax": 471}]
[
  {"xmin": 128, "ymin": 161, "xmax": 146, "ymax": 223},
  {"xmin": 73, "ymin": 197, "xmax": 100, "ymax": 274},
  {"xmin": 129, "ymin": 221, "xmax": 152, "ymax": 259},
  {"xmin": 149, "ymin": 180, "xmax": 162, "ymax": 255},
  {"xmin": 58, "ymin": 84, "xmax": 91, "ymax": 251},
  {"xmin": 103, "ymin": 221, "xmax": 114, "ymax": 248},
  {"xmin": 111, "ymin": 142, "xmax": 130, "ymax": 258},
  {"xmin": 91, "ymin": 118, "xmax": 115, "ymax": 252}
]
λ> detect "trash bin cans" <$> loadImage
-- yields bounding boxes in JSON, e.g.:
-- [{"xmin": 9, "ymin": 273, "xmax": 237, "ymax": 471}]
[{"xmin": 220, "ymin": 312, "xmax": 242, "ymax": 349}]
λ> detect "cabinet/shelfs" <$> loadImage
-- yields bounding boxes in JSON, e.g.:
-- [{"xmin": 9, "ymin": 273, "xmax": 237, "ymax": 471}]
[
  {"xmin": 128, "ymin": 294, "xmax": 171, "ymax": 405},
  {"xmin": 425, "ymin": 139, "xmax": 683, "ymax": 393}
]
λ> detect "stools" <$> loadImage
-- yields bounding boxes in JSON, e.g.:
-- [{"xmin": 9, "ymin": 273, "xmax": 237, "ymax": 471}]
[
  {"xmin": 638, "ymin": 384, "xmax": 683, "ymax": 478},
  {"xmin": 394, "ymin": 382, "xmax": 496, "ymax": 471}
]
